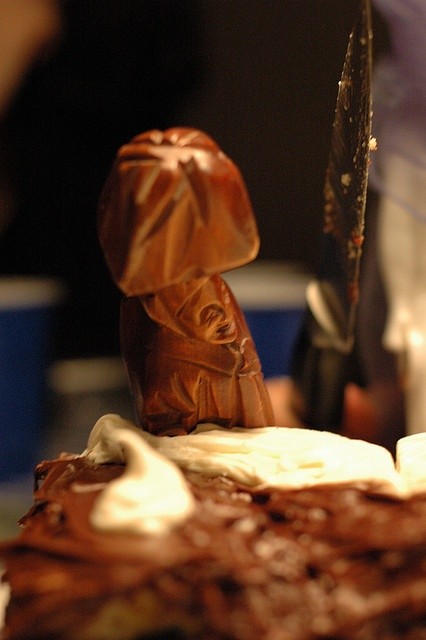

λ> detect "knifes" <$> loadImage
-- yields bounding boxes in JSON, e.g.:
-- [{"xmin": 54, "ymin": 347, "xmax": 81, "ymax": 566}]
[{"xmin": 297, "ymin": 5, "xmax": 374, "ymax": 440}]
